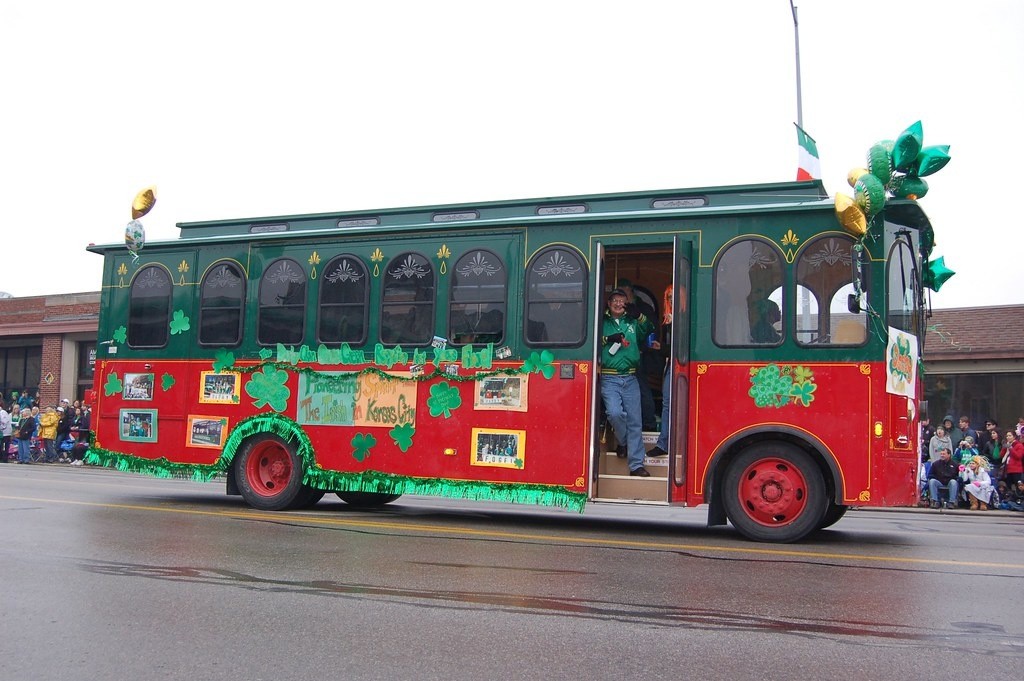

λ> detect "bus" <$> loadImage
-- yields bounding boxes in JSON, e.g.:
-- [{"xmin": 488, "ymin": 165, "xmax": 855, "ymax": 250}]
[{"xmin": 86, "ymin": 177, "xmax": 932, "ymax": 545}]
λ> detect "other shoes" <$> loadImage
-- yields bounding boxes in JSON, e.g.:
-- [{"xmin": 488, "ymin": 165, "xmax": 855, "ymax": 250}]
[
  {"xmin": 948, "ymin": 503, "xmax": 956, "ymax": 508},
  {"xmin": 70, "ymin": 459, "xmax": 84, "ymax": 466},
  {"xmin": 646, "ymin": 446, "xmax": 667, "ymax": 457},
  {"xmin": 617, "ymin": 444, "xmax": 627, "ymax": 458},
  {"xmin": 642, "ymin": 426, "xmax": 657, "ymax": 432},
  {"xmin": 930, "ymin": 502, "xmax": 940, "ymax": 508},
  {"xmin": 630, "ymin": 467, "xmax": 650, "ymax": 476}
]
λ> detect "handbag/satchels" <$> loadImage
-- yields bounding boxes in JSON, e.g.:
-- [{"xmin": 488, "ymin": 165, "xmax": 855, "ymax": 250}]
[{"xmin": 14, "ymin": 429, "xmax": 20, "ymax": 438}]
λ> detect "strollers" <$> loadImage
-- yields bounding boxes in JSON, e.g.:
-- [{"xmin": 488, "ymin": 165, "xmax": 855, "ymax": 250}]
[{"xmin": 7, "ymin": 437, "xmax": 43, "ymax": 463}]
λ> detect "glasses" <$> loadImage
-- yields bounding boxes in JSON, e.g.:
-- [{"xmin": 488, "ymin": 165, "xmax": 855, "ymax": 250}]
[
  {"xmin": 986, "ymin": 424, "xmax": 992, "ymax": 426},
  {"xmin": 610, "ymin": 298, "xmax": 625, "ymax": 304}
]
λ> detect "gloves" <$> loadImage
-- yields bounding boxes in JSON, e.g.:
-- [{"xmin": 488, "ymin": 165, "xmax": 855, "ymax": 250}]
[
  {"xmin": 608, "ymin": 332, "xmax": 625, "ymax": 343},
  {"xmin": 622, "ymin": 302, "xmax": 640, "ymax": 319}
]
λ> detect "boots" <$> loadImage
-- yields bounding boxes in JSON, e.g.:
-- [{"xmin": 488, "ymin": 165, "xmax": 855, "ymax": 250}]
[
  {"xmin": 980, "ymin": 501, "xmax": 987, "ymax": 510},
  {"xmin": 969, "ymin": 495, "xmax": 979, "ymax": 510}
]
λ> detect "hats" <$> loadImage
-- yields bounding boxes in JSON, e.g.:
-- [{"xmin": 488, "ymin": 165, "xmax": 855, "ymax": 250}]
[
  {"xmin": 55, "ymin": 407, "xmax": 64, "ymax": 412},
  {"xmin": 617, "ymin": 278, "xmax": 632, "ymax": 287},
  {"xmin": 997, "ymin": 481, "xmax": 1006, "ymax": 487},
  {"xmin": 608, "ymin": 289, "xmax": 626, "ymax": 298},
  {"xmin": 12, "ymin": 392, "xmax": 19, "ymax": 396},
  {"xmin": 61, "ymin": 399, "xmax": 69, "ymax": 403}
]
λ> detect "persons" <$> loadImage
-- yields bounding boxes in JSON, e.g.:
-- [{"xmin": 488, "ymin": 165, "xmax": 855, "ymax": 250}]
[
  {"xmin": 597, "ymin": 288, "xmax": 657, "ymax": 478},
  {"xmin": 750, "ymin": 300, "xmax": 804, "ymax": 344},
  {"xmin": 646, "ymin": 283, "xmax": 688, "ymax": 459},
  {"xmin": 916, "ymin": 414, "xmax": 1024, "ymax": 513},
  {"xmin": 0, "ymin": 382, "xmax": 91, "ymax": 466},
  {"xmin": 612, "ymin": 278, "xmax": 663, "ymax": 433}
]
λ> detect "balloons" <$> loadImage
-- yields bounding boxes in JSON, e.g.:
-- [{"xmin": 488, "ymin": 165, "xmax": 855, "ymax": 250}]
[
  {"xmin": 124, "ymin": 185, "xmax": 159, "ymax": 253},
  {"xmin": 830, "ymin": 117, "xmax": 952, "ymax": 238},
  {"xmin": 919, "ymin": 255, "xmax": 957, "ymax": 294}
]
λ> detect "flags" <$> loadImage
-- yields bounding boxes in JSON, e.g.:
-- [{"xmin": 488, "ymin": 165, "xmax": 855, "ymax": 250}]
[{"xmin": 795, "ymin": 126, "xmax": 822, "ymax": 182}]
